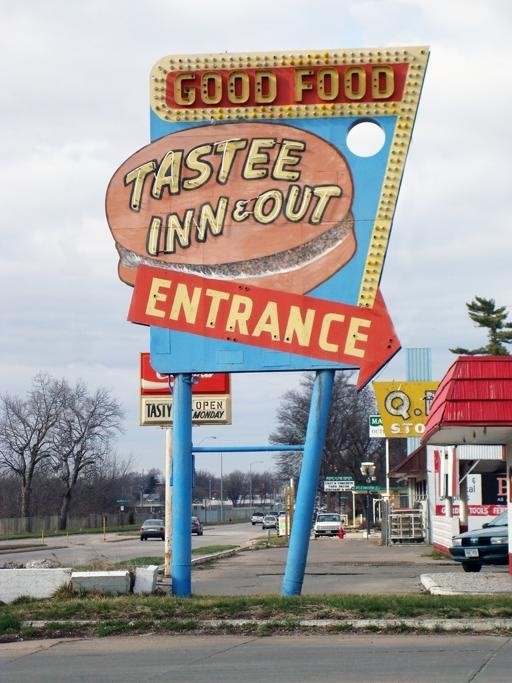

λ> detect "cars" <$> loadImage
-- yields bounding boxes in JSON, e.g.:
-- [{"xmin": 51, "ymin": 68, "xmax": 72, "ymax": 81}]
[
  {"xmin": 191, "ymin": 516, "xmax": 203, "ymax": 535},
  {"xmin": 448, "ymin": 508, "xmax": 508, "ymax": 572},
  {"xmin": 313, "ymin": 513, "xmax": 342, "ymax": 539},
  {"xmin": 251, "ymin": 511, "xmax": 286, "ymax": 529},
  {"xmin": 140, "ymin": 519, "xmax": 165, "ymax": 541}
]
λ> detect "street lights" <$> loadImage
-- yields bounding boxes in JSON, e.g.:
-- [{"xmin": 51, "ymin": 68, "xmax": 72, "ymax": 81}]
[
  {"xmin": 249, "ymin": 460, "xmax": 264, "ymax": 507},
  {"xmin": 359, "ymin": 462, "xmax": 377, "ymax": 535},
  {"xmin": 197, "ymin": 435, "xmax": 217, "ymax": 447}
]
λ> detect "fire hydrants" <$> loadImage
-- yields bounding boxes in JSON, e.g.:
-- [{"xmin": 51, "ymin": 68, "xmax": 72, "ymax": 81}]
[{"xmin": 338, "ymin": 527, "xmax": 345, "ymax": 539}]
